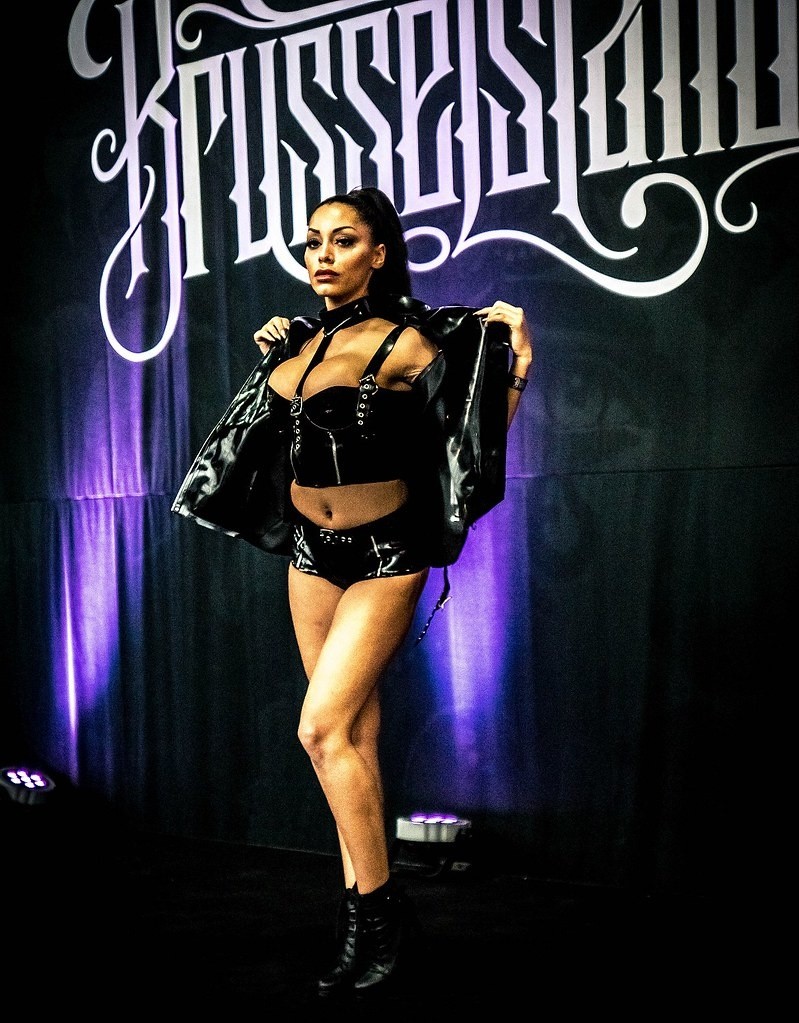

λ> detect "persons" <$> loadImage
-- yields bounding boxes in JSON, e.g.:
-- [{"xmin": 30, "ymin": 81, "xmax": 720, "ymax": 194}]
[{"xmin": 253, "ymin": 186, "xmax": 532, "ymax": 996}]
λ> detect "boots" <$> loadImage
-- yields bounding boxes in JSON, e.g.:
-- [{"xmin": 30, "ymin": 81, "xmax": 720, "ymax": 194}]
[
  {"xmin": 318, "ymin": 879, "xmax": 363, "ymax": 995},
  {"xmin": 351, "ymin": 881, "xmax": 410, "ymax": 989}
]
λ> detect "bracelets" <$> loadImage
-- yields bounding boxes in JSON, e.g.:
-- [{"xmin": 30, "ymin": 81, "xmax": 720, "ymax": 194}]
[{"xmin": 507, "ymin": 372, "xmax": 528, "ymax": 392}]
